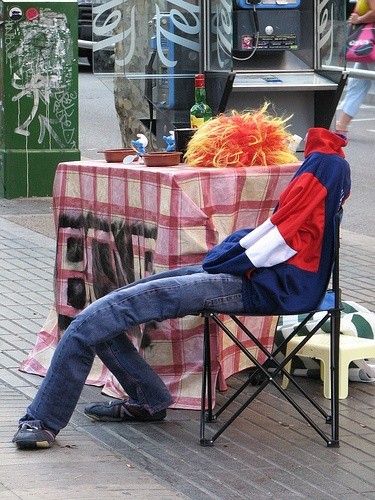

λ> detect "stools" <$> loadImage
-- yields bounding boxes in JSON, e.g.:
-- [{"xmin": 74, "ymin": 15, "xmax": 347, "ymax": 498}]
[{"xmin": 283, "ymin": 333, "xmax": 375, "ymax": 398}]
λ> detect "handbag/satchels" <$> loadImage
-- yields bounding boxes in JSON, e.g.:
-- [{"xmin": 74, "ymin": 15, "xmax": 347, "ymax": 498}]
[{"xmin": 336, "ymin": 20, "xmax": 375, "ymax": 63}]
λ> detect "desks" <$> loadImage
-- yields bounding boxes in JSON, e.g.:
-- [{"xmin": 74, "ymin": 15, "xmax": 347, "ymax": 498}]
[{"xmin": 18, "ymin": 157, "xmax": 305, "ymax": 412}]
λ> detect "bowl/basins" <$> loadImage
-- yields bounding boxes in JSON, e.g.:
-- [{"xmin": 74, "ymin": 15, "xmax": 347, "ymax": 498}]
[
  {"xmin": 141, "ymin": 152, "xmax": 184, "ymax": 167},
  {"xmin": 98, "ymin": 149, "xmax": 140, "ymax": 163}
]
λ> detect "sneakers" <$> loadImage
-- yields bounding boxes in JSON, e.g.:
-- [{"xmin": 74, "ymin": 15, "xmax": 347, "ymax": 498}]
[
  {"xmin": 12, "ymin": 422, "xmax": 56, "ymax": 448},
  {"xmin": 82, "ymin": 398, "xmax": 167, "ymax": 422}
]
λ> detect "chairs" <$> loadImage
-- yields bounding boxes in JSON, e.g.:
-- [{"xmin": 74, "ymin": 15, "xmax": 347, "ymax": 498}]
[{"xmin": 199, "ymin": 206, "xmax": 344, "ymax": 448}]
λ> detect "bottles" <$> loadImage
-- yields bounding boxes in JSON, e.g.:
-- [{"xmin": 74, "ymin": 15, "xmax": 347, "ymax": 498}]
[{"xmin": 189, "ymin": 74, "xmax": 212, "ymax": 129}]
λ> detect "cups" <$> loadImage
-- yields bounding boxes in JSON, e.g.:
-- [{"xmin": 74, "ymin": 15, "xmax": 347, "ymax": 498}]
[{"xmin": 174, "ymin": 128, "xmax": 199, "ymax": 163}]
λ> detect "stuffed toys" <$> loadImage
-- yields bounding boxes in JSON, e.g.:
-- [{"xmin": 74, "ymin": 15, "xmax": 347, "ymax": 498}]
[
  {"xmin": 131, "ymin": 134, "xmax": 148, "ymax": 155},
  {"xmin": 163, "ymin": 131, "xmax": 175, "ymax": 151}
]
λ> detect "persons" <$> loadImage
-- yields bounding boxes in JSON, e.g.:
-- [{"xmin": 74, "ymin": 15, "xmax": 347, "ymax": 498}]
[
  {"xmin": 13, "ymin": 128, "xmax": 351, "ymax": 449},
  {"xmin": 333, "ymin": 0, "xmax": 375, "ymax": 135}
]
